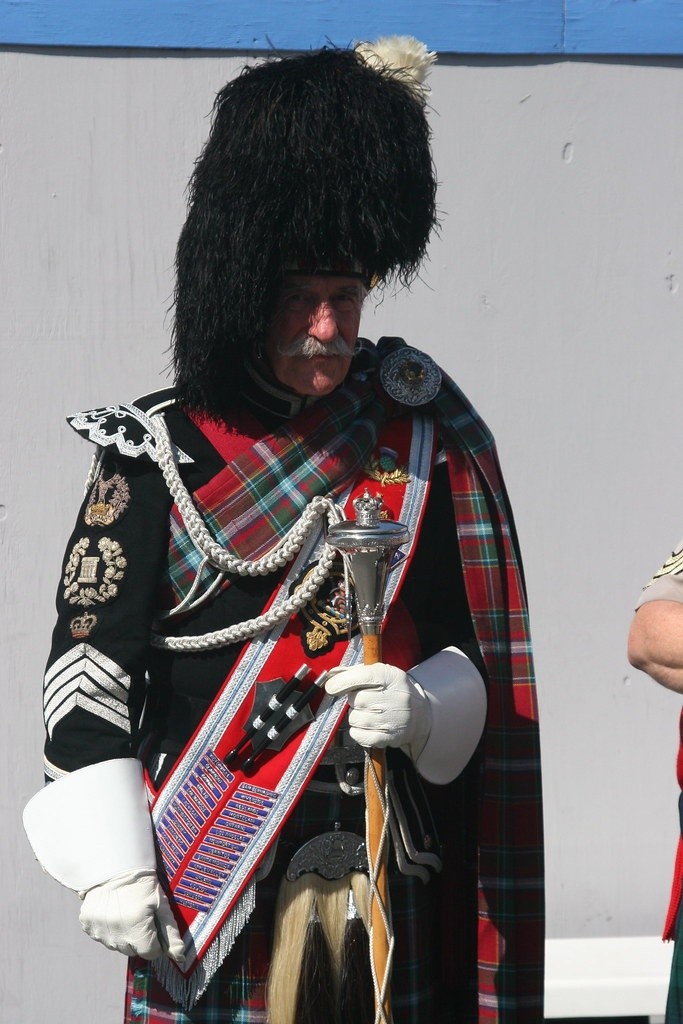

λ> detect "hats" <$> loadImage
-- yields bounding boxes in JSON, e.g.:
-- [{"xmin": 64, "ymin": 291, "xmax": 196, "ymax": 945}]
[{"xmin": 169, "ymin": 33, "xmax": 439, "ymax": 394}]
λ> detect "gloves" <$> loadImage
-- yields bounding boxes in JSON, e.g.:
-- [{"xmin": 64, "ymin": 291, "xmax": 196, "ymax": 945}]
[
  {"xmin": 325, "ymin": 645, "xmax": 487, "ymax": 785},
  {"xmin": 20, "ymin": 760, "xmax": 186, "ymax": 964}
]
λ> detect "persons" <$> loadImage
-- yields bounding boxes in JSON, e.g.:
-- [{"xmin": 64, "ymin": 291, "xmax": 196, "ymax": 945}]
[
  {"xmin": 23, "ymin": 35, "xmax": 547, "ymax": 1024},
  {"xmin": 628, "ymin": 535, "xmax": 683, "ymax": 1024}
]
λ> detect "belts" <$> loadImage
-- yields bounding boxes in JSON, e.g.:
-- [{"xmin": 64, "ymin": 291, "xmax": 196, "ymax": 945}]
[{"xmin": 306, "ymin": 758, "xmax": 365, "ymax": 794}]
